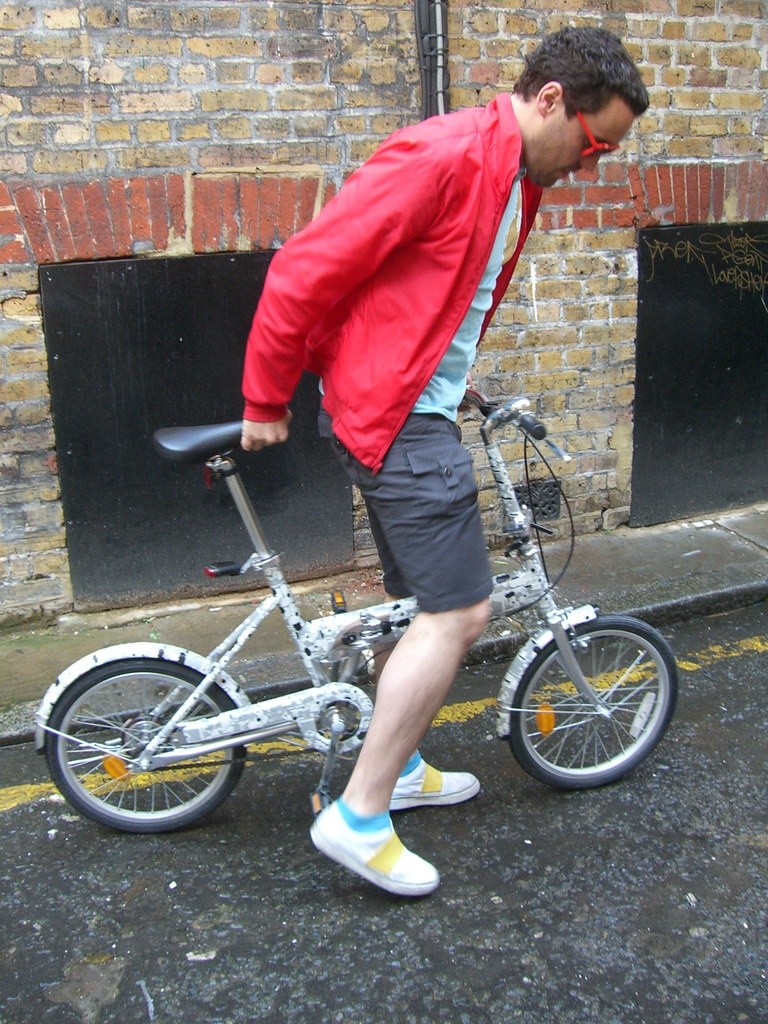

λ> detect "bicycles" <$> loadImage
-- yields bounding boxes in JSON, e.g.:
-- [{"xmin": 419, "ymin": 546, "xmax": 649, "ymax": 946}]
[{"xmin": 33, "ymin": 388, "xmax": 684, "ymax": 833}]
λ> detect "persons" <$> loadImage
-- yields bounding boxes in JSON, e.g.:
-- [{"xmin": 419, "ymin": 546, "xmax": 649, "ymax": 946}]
[{"xmin": 241, "ymin": 25, "xmax": 652, "ymax": 893}]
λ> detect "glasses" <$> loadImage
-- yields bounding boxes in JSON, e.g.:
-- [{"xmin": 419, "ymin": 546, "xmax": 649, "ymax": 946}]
[{"xmin": 545, "ymin": 78, "xmax": 620, "ymax": 160}]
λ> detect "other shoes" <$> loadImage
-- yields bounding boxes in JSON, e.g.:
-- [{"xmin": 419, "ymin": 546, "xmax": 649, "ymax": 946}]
[
  {"xmin": 389, "ymin": 759, "xmax": 482, "ymax": 809},
  {"xmin": 310, "ymin": 801, "xmax": 440, "ymax": 895}
]
